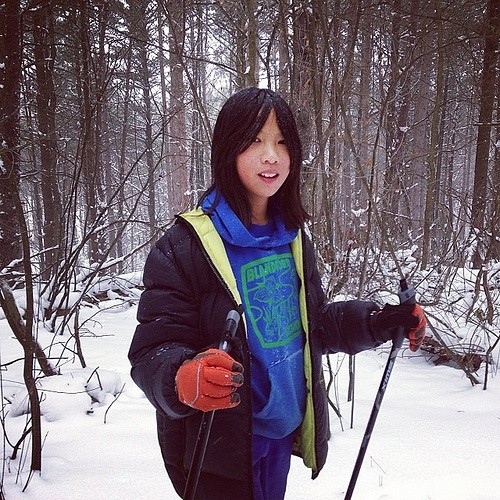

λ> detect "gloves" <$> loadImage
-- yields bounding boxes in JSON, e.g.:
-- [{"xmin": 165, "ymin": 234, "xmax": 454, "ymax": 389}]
[
  {"xmin": 174, "ymin": 338, "xmax": 245, "ymax": 412},
  {"xmin": 373, "ymin": 302, "xmax": 428, "ymax": 351}
]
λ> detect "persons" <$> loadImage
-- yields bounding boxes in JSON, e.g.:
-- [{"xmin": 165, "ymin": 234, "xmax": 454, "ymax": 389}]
[{"xmin": 129, "ymin": 87, "xmax": 428, "ymax": 500}]
